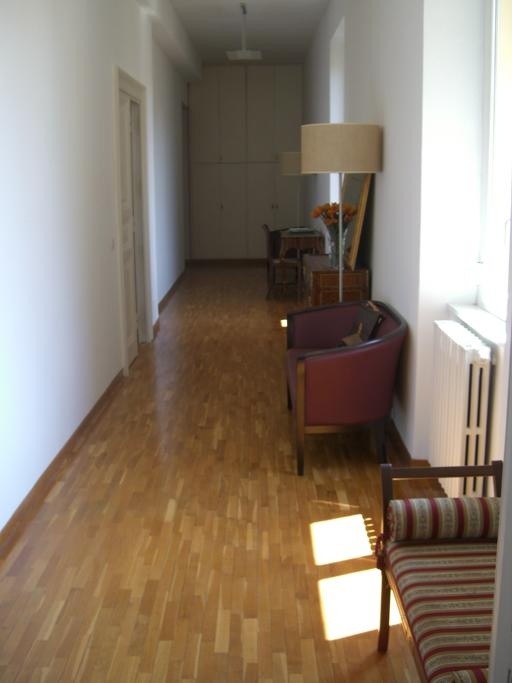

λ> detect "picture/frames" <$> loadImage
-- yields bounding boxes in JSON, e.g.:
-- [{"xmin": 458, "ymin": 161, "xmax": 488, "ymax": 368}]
[{"xmin": 343, "ymin": 173, "xmax": 371, "ymax": 272}]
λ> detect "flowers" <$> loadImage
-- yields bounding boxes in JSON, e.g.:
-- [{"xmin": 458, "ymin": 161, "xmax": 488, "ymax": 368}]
[{"xmin": 310, "ymin": 201, "xmax": 357, "ymax": 265}]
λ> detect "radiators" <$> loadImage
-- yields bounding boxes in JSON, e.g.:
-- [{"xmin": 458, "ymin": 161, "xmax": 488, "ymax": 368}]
[{"xmin": 428, "ymin": 320, "xmax": 491, "ymax": 498}]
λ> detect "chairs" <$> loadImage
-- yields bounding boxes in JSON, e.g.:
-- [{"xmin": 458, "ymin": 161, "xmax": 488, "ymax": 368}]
[
  {"xmin": 287, "ymin": 301, "xmax": 409, "ymax": 476},
  {"xmin": 263, "ymin": 224, "xmax": 303, "ymax": 304}
]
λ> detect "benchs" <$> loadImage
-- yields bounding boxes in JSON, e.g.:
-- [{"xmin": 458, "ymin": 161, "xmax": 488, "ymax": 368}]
[{"xmin": 378, "ymin": 461, "xmax": 503, "ymax": 683}]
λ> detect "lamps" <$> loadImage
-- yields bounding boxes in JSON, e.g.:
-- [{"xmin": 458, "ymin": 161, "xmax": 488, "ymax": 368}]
[
  {"xmin": 301, "ymin": 124, "xmax": 383, "ymax": 303},
  {"xmin": 279, "ymin": 152, "xmax": 301, "ymax": 228}
]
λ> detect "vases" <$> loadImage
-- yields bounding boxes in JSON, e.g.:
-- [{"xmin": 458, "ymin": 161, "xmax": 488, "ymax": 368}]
[{"xmin": 330, "ymin": 233, "xmax": 345, "ymax": 271}]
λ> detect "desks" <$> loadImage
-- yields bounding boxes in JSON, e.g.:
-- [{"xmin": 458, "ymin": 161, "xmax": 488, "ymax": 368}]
[{"xmin": 275, "ymin": 230, "xmax": 325, "ymax": 297}]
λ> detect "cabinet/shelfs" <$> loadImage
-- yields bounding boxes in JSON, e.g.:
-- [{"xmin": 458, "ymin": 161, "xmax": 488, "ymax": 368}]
[
  {"xmin": 189, "ymin": 64, "xmax": 304, "ymax": 260},
  {"xmin": 303, "ymin": 254, "xmax": 369, "ymax": 307}
]
